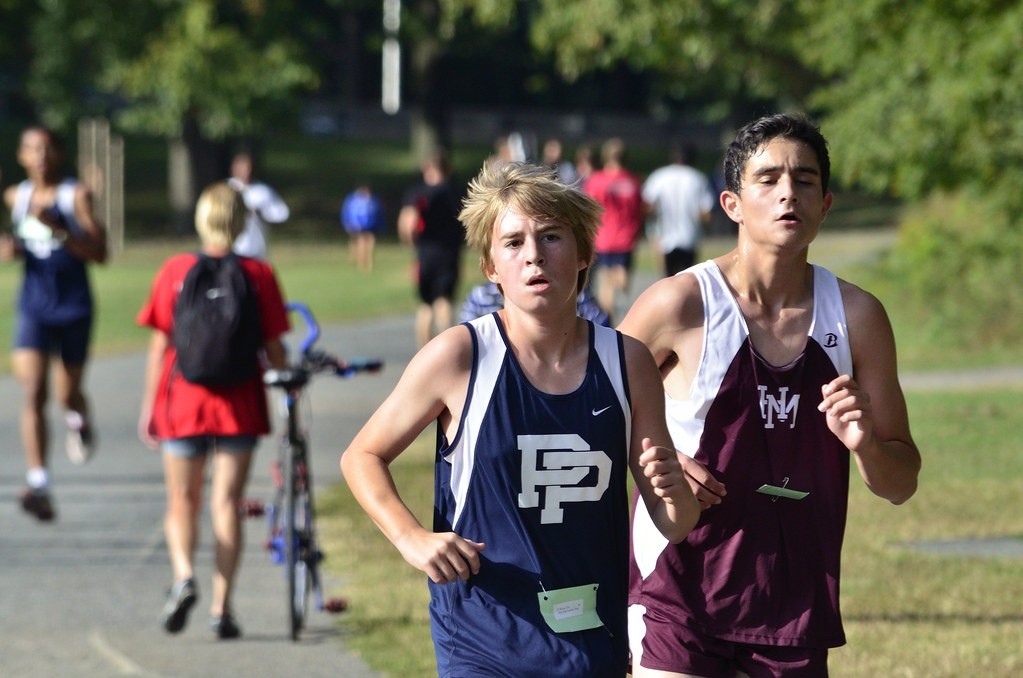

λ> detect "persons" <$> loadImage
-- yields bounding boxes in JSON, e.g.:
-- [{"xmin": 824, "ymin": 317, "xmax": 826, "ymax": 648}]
[
  {"xmin": 0, "ymin": 121, "xmax": 109, "ymax": 519},
  {"xmin": 612, "ymin": 113, "xmax": 922, "ymax": 678},
  {"xmin": 583, "ymin": 136, "xmax": 647, "ymax": 315},
  {"xmin": 639, "ymin": 135, "xmax": 715, "ymax": 278},
  {"xmin": 459, "ymin": 276, "xmax": 618, "ymax": 334},
  {"xmin": 226, "ymin": 152, "xmax": 294, "ymax": 267},
  {"xmin": 336, "ymin": 155, "xmax": 702, "ymax": 678},
  {"xmin": 491, "ymin": 130, "xmax": 604, "ymax": 194},
  {"xmin": 397, "ymin": 155, "xmax": 468, "ymax": 353},
  {"xmin": 132, "ymin": 181, "xmax": 296, "ymax": 640}
]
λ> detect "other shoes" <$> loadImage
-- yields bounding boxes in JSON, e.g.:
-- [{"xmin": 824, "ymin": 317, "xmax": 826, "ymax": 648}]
[
  {"xmin": 22, "ymin": 486, "xmax": 56, "ymax": 523},
  {"xmin": 210, "ymin": 614, "xmax": 240, "ymax": 638},
  {"xmin": 160, "ymin": 583, "xmax": 200, "ymax": 638},
  {"xmin": 65, "ymin": 404, "xmax": 95, "ymax": 466}
]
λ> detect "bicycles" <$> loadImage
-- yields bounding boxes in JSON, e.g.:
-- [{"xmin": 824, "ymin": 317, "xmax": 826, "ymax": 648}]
[{"xmin": 237, "ymin": 303, "xmax": 385, "ymax": 642}]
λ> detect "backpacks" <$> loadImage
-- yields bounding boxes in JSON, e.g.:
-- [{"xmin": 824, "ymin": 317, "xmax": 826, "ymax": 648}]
[{"xmin": 173, "ymin": 248, "xmax": 269, "ymax": 390}]
